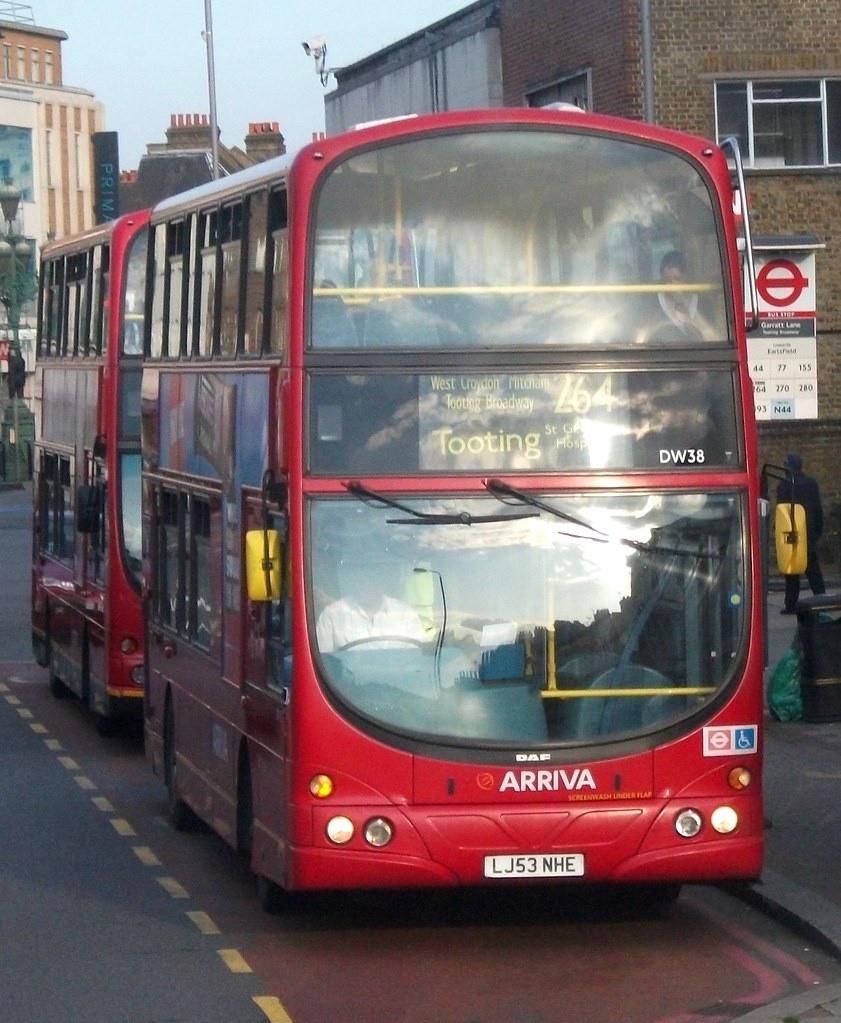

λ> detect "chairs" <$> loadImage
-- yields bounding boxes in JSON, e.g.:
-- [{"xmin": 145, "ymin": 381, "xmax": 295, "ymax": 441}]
[
  {"xmin": 537, "ymin": 638, "xmax": 682, "ymax": 739},
  {"xmin": 312, "ymin": 279, "xmax": 480, "ymax": 345}
]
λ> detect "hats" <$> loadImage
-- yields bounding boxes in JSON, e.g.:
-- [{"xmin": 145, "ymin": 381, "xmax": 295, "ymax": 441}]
[{"xmin": 784, "ymin": 455, "xmax": 802, "ymax": 467}]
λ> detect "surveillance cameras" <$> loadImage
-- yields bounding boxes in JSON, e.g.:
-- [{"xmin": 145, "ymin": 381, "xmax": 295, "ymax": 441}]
[{"xmin": 300, "ymin": 37, "xmax": 325, "ymax": 57}]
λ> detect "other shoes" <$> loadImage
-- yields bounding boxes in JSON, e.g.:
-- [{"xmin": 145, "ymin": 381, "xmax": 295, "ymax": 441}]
[{"xmin": 780, "ymin": 605, "xmax": 796, "ymax": 614}]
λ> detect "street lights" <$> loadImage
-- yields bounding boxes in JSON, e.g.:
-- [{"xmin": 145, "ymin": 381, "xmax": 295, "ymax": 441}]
[{"xmin": 0, "ymin": 178, "xmax": 25, "ymax": 487}]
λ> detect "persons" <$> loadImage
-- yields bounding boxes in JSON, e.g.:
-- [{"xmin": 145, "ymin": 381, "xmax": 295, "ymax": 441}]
[
  {"xmin": 642, "ymin": 251, "xmax": 716, "ymax": 340},
  {"xmin": 315, "ymin": 563, "xmax": 426, "ymax": 652},
  {"xmin": 775, "ymin": 455, "xmax": 826, "ymax": 614}
]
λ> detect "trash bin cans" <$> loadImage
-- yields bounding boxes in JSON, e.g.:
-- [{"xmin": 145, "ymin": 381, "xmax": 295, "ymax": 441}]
[{"xmin": 796, "ymin": 594, "xmax": 841, "ymax": 724}]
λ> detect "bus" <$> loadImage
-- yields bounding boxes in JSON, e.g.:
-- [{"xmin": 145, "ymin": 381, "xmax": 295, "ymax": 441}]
[
  {"xmin": 28, "ymin": 209, "xmax": 151, "ymax": 727},
  {"xmin": 138, "ymin": 100, "xmax": 809, "ymax": 917}
]
